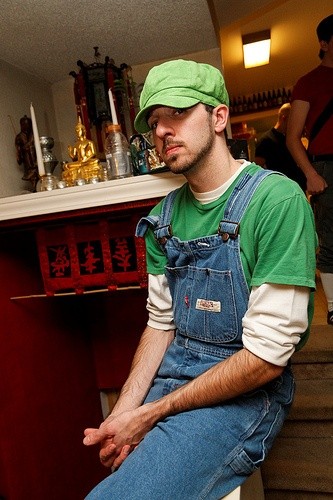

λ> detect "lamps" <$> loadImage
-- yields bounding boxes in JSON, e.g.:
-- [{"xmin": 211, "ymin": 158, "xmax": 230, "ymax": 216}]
[{"xmin": 241, "ymin": 28, "xmax": 271, "ymax": 69}]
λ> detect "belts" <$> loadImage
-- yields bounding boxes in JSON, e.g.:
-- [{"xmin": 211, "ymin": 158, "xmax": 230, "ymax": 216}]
[{"xmin": 309, "ymin": 153, "xmax": 333, "ymax": 161}]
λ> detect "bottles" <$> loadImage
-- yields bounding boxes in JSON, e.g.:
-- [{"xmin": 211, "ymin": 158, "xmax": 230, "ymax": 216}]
[{"xmin": 105, "ymin": 124, "xmax": 133, "ymax": 180}]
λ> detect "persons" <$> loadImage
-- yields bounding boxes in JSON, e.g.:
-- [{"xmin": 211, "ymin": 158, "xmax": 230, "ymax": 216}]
[
  {"xmin": 82, "ymin": 57, "xmax": 318, "ymax": 500},
  {"xmin": 63, "ymin": 116, "xmax": 100, "ymax": 168},
  {"xmin": 15, "ymin": 115, "xmax": 39, "ymax": 192},
  {"xmin": 257, "ymin": 103, "xmax": 310, "ymax": 199},
  {"xmin": 284, "ymin": 15, "xmax": 333, "ymax": 323}
]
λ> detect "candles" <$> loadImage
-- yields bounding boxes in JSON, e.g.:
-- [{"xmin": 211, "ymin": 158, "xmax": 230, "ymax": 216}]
[
  {"xmin": 30, "ymin": 102, "xmax": 45, "ymax": 178},
  {"xmin": 107, "ymin": 87, "xmax": 124, "ymax": 154}
]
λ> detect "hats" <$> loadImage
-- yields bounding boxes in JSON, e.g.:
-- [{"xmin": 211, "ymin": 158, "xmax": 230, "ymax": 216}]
[{"xmin": 133, "ymin": 59, "xmax": 229, "ymax": 134}]
